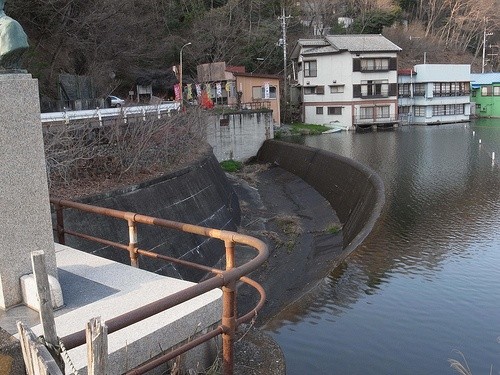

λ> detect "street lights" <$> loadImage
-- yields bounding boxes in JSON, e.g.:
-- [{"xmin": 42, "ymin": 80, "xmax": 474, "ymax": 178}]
[{"xmin": 180, "ymin": 42, "xmax": 192, "ymax": 104}]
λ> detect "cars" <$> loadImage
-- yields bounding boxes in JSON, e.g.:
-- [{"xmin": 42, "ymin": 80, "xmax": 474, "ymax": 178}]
[{"xmin": 110, "ymin": 96, "xmax": 125, "ymax": 108}]
